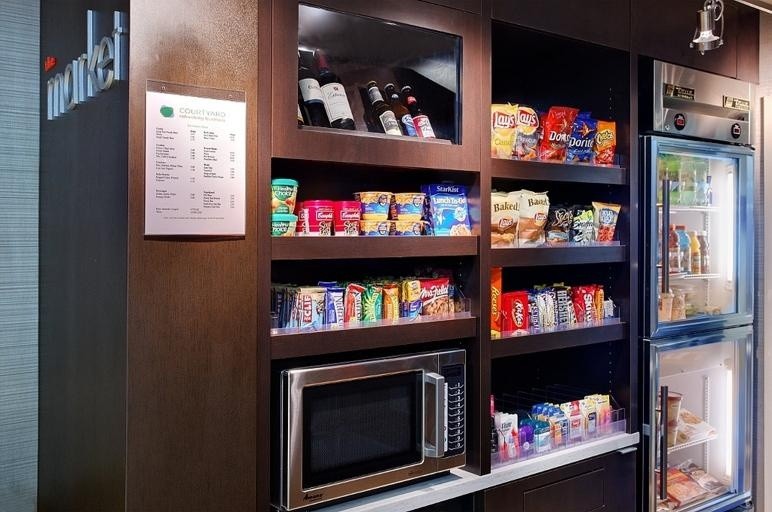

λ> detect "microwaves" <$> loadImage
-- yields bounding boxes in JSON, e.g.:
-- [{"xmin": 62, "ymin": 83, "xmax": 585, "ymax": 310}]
[{"xmin": 273, "ymin": 348, "xmax": 471, "ymax": 512}]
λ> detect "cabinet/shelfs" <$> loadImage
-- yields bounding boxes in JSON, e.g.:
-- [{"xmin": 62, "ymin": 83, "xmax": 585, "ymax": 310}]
[{"xmin": 258, "ymin": 0, "xmax": 772, "ymax": 511}]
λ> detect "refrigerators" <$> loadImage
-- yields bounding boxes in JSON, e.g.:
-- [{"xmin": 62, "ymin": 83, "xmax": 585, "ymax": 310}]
[{"xmin": 642, "ymin": 60, "xmax": 754, "ymax": 512}]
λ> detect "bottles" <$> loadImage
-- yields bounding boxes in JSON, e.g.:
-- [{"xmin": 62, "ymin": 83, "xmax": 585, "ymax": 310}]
[
  {"xmin": 297, "ymin": 47, "xmax": 436, "ymax": 139},
  {"xmin": 658, "ymin": 154, "xmax": 714, "ymax": 276}
]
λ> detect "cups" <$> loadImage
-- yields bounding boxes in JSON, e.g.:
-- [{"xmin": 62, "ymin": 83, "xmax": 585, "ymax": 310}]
[{"xmin": 656, "ymin": 294, "xmax": 686, "ymax": 321}]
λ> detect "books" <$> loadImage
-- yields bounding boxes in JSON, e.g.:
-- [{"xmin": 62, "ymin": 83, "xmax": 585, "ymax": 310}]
[
  {"xmin": 658, "ymin": 463, "xmax": 726, "ymax": 509},
  {"xmin": 677, "ymin": 407, "xmax": 715, "ymax": 443}
]
